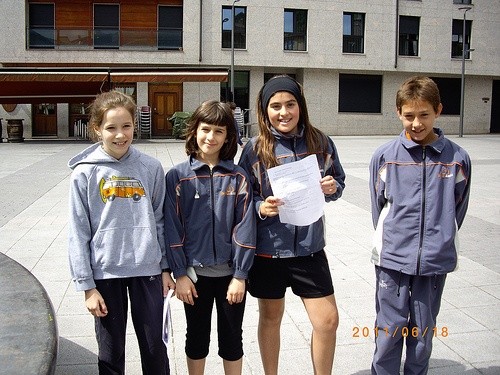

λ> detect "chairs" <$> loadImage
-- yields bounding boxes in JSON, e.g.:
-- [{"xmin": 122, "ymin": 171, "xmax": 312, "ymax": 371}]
[
  {"xmin": 138, "ymin": 105, "xmax": 152, "ymax": 140},
  {"xmin": 232, "ymin": 107, "xmax": 245, "ymax": 139}
]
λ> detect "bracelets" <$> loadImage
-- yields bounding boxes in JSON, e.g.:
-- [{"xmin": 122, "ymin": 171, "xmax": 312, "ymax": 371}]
[{"xmin": 258, "ymin": 202, "xmax": 268, "ymax": 221}]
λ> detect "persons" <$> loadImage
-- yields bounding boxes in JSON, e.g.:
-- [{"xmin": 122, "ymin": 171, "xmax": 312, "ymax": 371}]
[
  {"xmin": 66, "ymin": 90, "xmax": 176, "ymax": 375},
  {"xmin": 234, "ymin": 74, "xmax": 346, "ymax": 375},
  {"xmin": 369, "ymin": 75, "xmax": 472, "ymax": 375},
  {"xmin": 163, "ymin": 99, "xmax": 257, "ymax": 375}
]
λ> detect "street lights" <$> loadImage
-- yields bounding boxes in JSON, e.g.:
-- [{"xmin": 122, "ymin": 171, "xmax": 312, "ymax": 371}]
[
  {"xmin": 231, "ymin": 0, "xmax": 241, "ymax": 108},
  {"xmin": 458, "ymin": 7, "xmax": 472, "ymax": 138}
]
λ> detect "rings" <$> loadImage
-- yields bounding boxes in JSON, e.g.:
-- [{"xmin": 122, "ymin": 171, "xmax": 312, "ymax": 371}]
[{"xmin": 329, "ymin": 188, "xmax": 333, "ymax": 192}]
